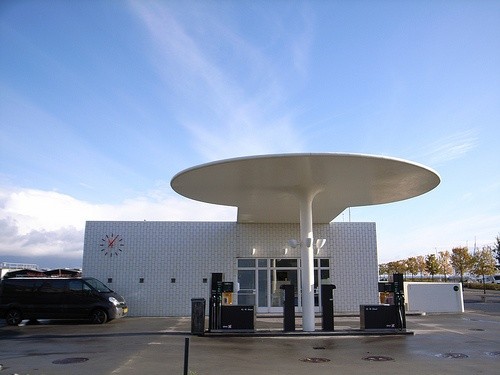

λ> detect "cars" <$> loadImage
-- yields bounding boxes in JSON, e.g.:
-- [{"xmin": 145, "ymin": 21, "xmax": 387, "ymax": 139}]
[{"xmin": 443, "ymin": 271, "xmax": 500, "ymax": 285}]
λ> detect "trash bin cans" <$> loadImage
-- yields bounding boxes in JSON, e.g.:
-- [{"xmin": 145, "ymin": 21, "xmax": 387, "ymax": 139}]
[{"xmin": 191, "ymin": 297, "xmax": 206, "ymax": 334}]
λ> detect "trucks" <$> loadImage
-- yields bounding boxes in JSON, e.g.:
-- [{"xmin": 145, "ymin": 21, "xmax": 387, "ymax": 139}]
[{"xmin": 0, "ymin": 277, "xmax": 127, "ymax": 324}]
